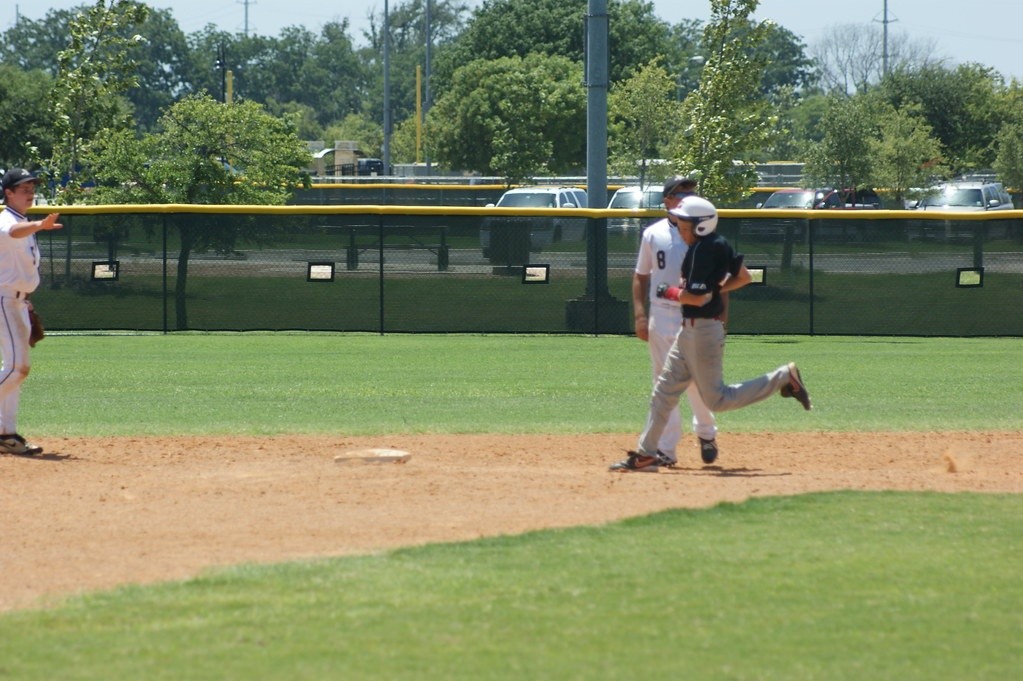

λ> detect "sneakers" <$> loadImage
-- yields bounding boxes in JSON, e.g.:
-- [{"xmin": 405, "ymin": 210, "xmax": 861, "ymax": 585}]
[
  {"xmin": 609, "ymin": 454, "xmax": 658, "ymax": 473},
  {"xmin": 0, "ymin": 434, "xmax": 42, "ymax": 456},
  {"xmin": 698, "ymin": 436, "xmax": 718, "ymax": 463},
  {"xmin": 781, "ymin": 361, "xmax": 810, "ymax": 412},
  {"xmin": 627, "ymin": 448, "xmax": 677, "ymax": 467}
]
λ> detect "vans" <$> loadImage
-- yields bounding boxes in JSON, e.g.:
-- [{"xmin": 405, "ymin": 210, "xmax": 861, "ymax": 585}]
[{"xmin": 478, "ymin": 184, "xmax": 1017, "ymax": 257}]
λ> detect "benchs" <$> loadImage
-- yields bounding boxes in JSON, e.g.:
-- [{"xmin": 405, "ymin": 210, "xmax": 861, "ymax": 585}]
[{"xmin": 314, "ymin": 223, "xmax": 452, "ymax": 269}]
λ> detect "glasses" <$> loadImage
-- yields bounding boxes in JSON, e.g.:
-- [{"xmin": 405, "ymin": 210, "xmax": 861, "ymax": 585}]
[{"xmin": 668, "ymin": 191, "xmax": 698, "ymax": 198}]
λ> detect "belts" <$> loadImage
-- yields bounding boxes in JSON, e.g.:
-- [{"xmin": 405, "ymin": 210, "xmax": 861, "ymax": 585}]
[
  {"xmin": 681, "ymin": 315, "xmax": 720, "ymax": 327},
  {"xmin": 16, "ymin": 290, "xmax": 30, "ymax": 299}
]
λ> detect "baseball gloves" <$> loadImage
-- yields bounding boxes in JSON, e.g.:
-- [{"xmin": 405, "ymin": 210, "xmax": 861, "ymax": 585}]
[{"xmin": 26, "ymin": 298, "xmax": 45, "ymax": 346}]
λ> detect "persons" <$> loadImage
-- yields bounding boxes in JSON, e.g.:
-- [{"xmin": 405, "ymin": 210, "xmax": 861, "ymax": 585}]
[
  {"xmin": 0, "ymin": 167, "xmax": 63, "ymax": 456},
  {"xmin": 633, "ymin": 174, "xmax": 728, "ymax": 466},
  {"xmin": 608, "ymin": 196, "xmax": 811, "ymax": 474}
]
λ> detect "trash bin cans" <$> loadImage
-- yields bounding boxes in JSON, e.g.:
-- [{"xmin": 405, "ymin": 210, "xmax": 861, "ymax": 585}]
[{"xmin": 491, "ymin": 220, "xmax": 530, "ymax": 274}]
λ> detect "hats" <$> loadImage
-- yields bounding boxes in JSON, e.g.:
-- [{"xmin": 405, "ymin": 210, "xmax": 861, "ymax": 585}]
[
  {"xmin": 2, "ymin": 168, "xmax": 42, "ymax": 190},
  {"xmin": 662, "ymin": 174, "xmax": 697, "ymax": 199}
]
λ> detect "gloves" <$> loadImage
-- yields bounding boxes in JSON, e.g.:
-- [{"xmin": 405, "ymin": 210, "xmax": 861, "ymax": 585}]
[{"xmin": 657, "ymin": 282, "xmax": 670, "ymax": 297}]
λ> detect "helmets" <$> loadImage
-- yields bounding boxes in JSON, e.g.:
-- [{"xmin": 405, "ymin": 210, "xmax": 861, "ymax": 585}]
[{"xmin": 667, "ymin": 195, "xmax": 719, "ymax": 237}]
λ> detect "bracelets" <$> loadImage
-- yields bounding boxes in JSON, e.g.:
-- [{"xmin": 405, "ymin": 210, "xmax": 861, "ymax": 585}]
[{"xmin": 635, "ymin": 314, "xmax": 646, "ymax": 320}]
